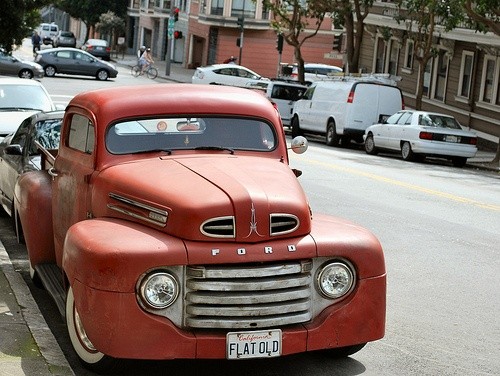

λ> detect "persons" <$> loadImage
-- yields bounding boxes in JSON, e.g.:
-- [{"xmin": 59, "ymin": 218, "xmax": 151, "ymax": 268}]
[
  {"xmin": 137, "ymin": 45, "xmax": 154, "ymax": 75},
  {"xmin": 32, "ymin": 30, "xmax": 41, "ymax": 54}
]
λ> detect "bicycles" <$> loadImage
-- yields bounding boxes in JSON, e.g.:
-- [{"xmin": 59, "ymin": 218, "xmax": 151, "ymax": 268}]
[{"xmin": 132, "ymin": 61, "xmax": 158, "ymax": 80}]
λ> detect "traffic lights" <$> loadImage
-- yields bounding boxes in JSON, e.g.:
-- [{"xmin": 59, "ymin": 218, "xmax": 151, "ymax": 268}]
[
  {"xmin": 175, "ymin": 31, "xmax": 182, "ymax": 39},
  {"xmin": 275, "ymin": 33, "xmax": 283, "ymax": 50},
  {"xmin": 333, "ymin": 33, "xmax": 342, "ymax": 52},
  {"xmin": 282, "ymin": 66, "xmax": 293, "ymax": 75},
  {"xmin": 173, "ymin": 7, "xmax": 179, "ymax": 21},
  {"xmin": 237, "ymin": 18, "xmax": 244, "ymax": 25}
]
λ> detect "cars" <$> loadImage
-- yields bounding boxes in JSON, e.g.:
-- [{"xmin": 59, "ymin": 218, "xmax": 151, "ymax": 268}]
[
  {"xmin": 54, "ymin": 31, "xmax": 76, "ymax": 48},
  {"xmin": 37, "ymin": 23, "xmax": 59, "ymax": 40},
  {"xmin": 0, "ymin": 78, "xmax": 57, "ymax": 143},
  {"xmin": 0, "ymin": 48, "xmax": 44, "ymax": 79},
  {"xmin": 0, "ymin": 109, "xmax": 150, "ymax": 245},
  {"xmin": 362, "ymin": 108, "xmax": 479, "ymax": 165},
  {"xmin": 82, "ymin": 38, "xmax": 111, "ymax": 61},
  {"xmin": 281, "ymin": 63, "xmax": 343, "ymax": 84},
  {"xmin": 34, "ymin": 47, "xmax": 118, "ymax": 81},
  {"xmin": 192, "ymin": 63, "xmax": 272, "ymax": 92},
  {"xmin": 14, "ymin": 82, "xmax": 387, "ymax": 372},
  {"xmin": 246, "ymin": 80, "xmax": 308, "ymax": 126}
]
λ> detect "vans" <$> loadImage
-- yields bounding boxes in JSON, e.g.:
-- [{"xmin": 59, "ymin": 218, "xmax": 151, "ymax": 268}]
[{"xmin": 290, "ymin": 79, "xmax": 405, "ymax": 146}]
